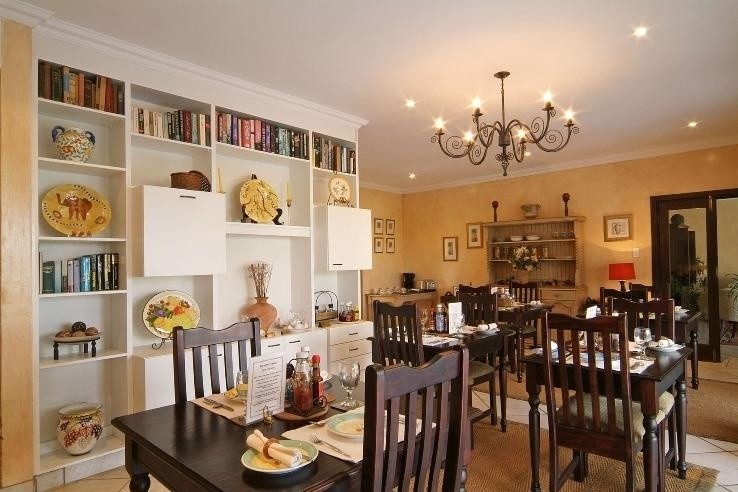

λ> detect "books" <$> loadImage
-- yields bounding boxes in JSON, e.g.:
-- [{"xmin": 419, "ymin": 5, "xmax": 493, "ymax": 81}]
[
  {"xmin": 38, "ymin": 61, "xmax": 124, "ymax": 116},
  {"xmin": 39, "ymin": 251, "xmax": 119, "ymax": 294},
  {"xmin": 132, "ymin": 105, "xmax": 357, "ymax": 175}
]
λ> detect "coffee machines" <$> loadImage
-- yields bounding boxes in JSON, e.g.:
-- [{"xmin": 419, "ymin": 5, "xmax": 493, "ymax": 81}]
[{"xmin": 403, "ymin": 272, "xmax": 415, "ymax": 292}]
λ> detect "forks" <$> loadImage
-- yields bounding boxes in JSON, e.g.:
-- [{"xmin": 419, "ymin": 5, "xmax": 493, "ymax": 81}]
[{"xmin": 312, "ymin": 435, "xmax": 351, "ymax": 458}]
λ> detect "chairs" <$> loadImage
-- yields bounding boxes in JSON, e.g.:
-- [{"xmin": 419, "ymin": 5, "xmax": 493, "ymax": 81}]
[
  {"xmin": 167, "ymin": 315, "xmax": 266, "ymax": 404},
  {"xmin": 698, "ymin": 270, "xmax": 737, "ymax": 339},
  {"xmin": 364, "ymin": 344, "xmax": 469, "ymax": 492},
  {"xmin": 518, "ymin": 283, "xmax": 701, "ymax": 492},
  {"xmin": 372, "ymin": 283, "xmax": 555, "ymax": 433}
]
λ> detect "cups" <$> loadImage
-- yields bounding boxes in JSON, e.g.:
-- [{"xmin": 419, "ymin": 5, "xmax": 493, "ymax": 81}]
[
  {"xmin": 456, "ymin": 323, "xmax": 500, "ymax": 337},
  {"xmin": 417, "ymin": 279, "xmax": 436, "ymax": 293},
  {"xmin": 371, "ymin": 286, "xmax": 407, "ymax": 295}
]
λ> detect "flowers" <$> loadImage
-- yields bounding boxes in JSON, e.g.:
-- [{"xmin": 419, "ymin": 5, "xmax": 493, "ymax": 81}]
[{"xmin": 507, "ymin": 244, "xmax": 542, "ymax": 272}]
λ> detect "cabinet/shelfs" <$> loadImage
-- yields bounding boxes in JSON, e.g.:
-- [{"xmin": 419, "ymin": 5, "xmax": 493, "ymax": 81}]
[
  {"xmin": 34, "ymin": 57, "xmax": 138, "ymax": 477},
  {"xmin": 254, "ymin": 326, "xmax": 328, "ymax": 374},
  {"xmin": 481, "ymin": 214, "xmax": 587, "ymax": 290},
  {"xmin": 311, "ymin": 129, "xmax": 376, "ymax": 323},
  {"xmin": 670, "ymin": 223, "xmax": 698, "ymax": 304},
  {"xmin": 211, "ymin": 102, "xmax": 315, "ymax": 331},
  {"xmin": 128, "ymin": 78, "xmax": 217, "ymax": 346},
  {"xmin": 323, "ymin": 319, "xmax": 375, "ymax": 384},
  {"xmin": 132, "ymin": 339, "xmax": 252, "ymax": 415}
]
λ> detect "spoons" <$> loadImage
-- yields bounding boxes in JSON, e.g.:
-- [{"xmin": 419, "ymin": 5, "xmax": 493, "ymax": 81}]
[{"xmin": 202, "ymin": 400, "xmax": 220, "ymax": 408}]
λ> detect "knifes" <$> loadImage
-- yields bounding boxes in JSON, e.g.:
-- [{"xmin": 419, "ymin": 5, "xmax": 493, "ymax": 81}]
[{"xmin": 204, "ymin": 397, "xmax": 234, "ymax": 412}]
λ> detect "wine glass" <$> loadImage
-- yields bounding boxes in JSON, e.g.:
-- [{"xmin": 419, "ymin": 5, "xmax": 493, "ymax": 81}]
[
  {"xmin": 633, "ymin": 327, "xmax": 652, "ymax": 360},
  {"xmin": 235, "ymin": 370, "xmax": 248, "ymax": 423},
  {"xmin": 337, "ymin": 359, "xmax": 361, "ymax": 408}
]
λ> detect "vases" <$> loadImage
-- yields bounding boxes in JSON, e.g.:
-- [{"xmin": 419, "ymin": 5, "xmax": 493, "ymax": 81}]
[
  {"xmin": 519, "ymin": 269, "xmax": 530, "ymax": 284},
  {"xmin": 49, "ymin": 123, "xmax": 98, "ymax": 164},
  {"xmin": 244, "ymin": 296, "xmax": 279, "ymax": 331}
]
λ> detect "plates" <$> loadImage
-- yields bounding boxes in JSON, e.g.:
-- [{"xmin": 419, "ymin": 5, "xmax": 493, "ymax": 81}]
[
  {"xmin": 495, "ymin": 234, "xmax": 541, "ymax": 241},
  {"xmin": 540, "ymin": 351, "xmax": 569, "ymax": 358},
  {"xmin": 49, "ymin": 332, "xmax": 103, "ymax": 342},
  {"xmin": 142, "ymin": 290, "xmax": 200, "ymax": 339},
  {"xmin": 675, "ymin": 309, "xmax": 690, "ymax": 314},
  {"xmin": 647, "ymin": 344, "xmax": 685, "ymax": 352},
  {"xmin": 241, "ymin": 440, "xmax": 318, "ymax": 475},
  {"xmin": 224, "ymin": 386, "xmax": 243, "ymax": 401},
  {"xmin": 240, "ymin": 180, "xmax": 281, "ymax": 223},
  {"xmin": 527, "ymin": 302, "xmax": 543, "ymax": 307},
  {"xmin": 42, "ymin": 184, "xmax": 112, "ymax": 236},
  {"xmin": 321, "ymin": 371, "xmax": 333, "ymax": 382},
  {"xmin": 330, "ymin": 176, "xmax": 352, "ymax": 204},
  {"xmin": 326, "ymin": 413, "xmax": 386, "ymax": 441}
]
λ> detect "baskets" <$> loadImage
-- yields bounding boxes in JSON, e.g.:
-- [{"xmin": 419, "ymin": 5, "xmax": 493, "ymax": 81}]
[{"xmin": 170, "ymin": 170, "xmax": 211, "ymax": 192}]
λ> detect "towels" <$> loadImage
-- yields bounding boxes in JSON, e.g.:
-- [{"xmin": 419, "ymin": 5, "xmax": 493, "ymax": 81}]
[{"xmin": 242, "ymin": 427, "xmax": 304, "ymax": 467}]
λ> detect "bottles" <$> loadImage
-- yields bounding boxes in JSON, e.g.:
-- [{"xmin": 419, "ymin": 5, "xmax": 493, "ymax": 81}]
[
  {"xmin": 521, "ymin": 201, "xmax": 539, "ymax": 218},
  {"xmin": 315, "ymin": 304, "xmax": 333, "ymax": 313},
  {"xmin": 339, "ymin": 301, "xmax": 359, "ymax": 322},
  {"xmin": 239, "ymin": 314, "xmax": 250, "ymax": 322},
  {"xmin": 595, "ymin": 337, "xmax": 603, "ymax": 353},
  {"xmin": 495, "ymin": 246, "xmax": 550, "ymax": 260},
  {"xmin": 420, "ymin": 304, "xmax": 449, "ymax": 334},
  {"xmin": 286, "ymin": 312, "xmax": 308, "ymax": 329},
  {"xmin": 552, "ymin": 232, "xmax": 574, "ymax": 239},
  {"xmin": 497, "ymin": 287, "xmax": 516, "ymax": 300},
  {"xmin": 612, "ymin": 339, "xmax": 620, "ymax": 353},
  {"xmin": 52, "ymin": 125, "xmax": 96, "ymax": 163},
  {"xmin": 287, "ymin": 347, "xmax": 324, "ymax": 412}
]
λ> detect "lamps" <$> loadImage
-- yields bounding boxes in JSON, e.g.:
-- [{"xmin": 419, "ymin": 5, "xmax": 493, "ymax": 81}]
[
  {"xmin": 428, "ymin": 69, "xmax": 581, "ymax": 179},
  {"xmin": 607, "ymin": 262, "xmax": 637, "ymax": 292}
]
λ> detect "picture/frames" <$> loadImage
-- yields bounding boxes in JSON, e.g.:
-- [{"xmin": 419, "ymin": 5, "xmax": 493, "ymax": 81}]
[
  {"xmin": 385, "ymin": 237, "xmax": 396, "ymax": 254},
  {"xmin": 385, "ymin": 218, "xmax": 396, "ymax": 235},
  {"xmin": 441, "ymin": 235, "xmax": 459, "ymax": 263},
  {"xmin": 464, "ymin": 221, "xmax": 484, "ymax": 251},
  {"xmin": 373, "ymin": 236, "xmax": 384, "ymax": 254},
  {"xmin": 373, "ymin": 217, "xmax": 384, "ymax": 235},
  {"xmin": 602, "ymin": 212, "xmax": 634, "ymax": 244}
]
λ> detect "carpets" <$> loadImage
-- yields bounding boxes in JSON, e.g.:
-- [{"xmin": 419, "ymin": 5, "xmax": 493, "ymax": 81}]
[
  {"xmin": 405, "ymin": 407, "xmax": 721, "ymax": 492},
  {"xmin": 470, "ymin": 354, "xmax": 738, "ymax": 444},
  {"xmin": 717, "ymin": 321, "xmax": 738, "ymax": 347}
]
